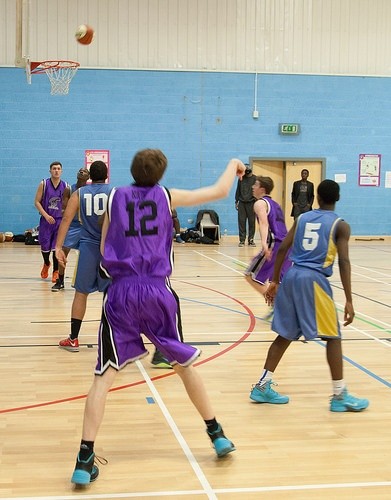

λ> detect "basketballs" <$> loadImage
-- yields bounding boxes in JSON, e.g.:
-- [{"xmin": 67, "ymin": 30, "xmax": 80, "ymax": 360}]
[{"xmin": 75, "ymin": 24, "xmax": 95, "ymax": 45}]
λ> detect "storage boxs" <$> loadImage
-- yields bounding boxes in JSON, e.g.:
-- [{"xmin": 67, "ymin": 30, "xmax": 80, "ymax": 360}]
[{"xmin": 24, "ymin": 230, "xmax": 40, "ymax": 245}]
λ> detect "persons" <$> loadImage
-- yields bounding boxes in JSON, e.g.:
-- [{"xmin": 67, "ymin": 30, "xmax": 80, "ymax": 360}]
[
  {"xmin": 35, "ymin": 162, "xmax": 69, "ymax": 283},
  {"xmin": 150, "ymin": 210, "xmax": 181, "ymax": 368},
  {"xmin": 235, "ymin": 164, "xmax": 258, "ymax": 246},
  {"xmin": 72, "ymin": 148, "xmax": 246, "ymax": 484},
  {"xmin": 250, "ymin": 180, "xmax": 369, "ymax": 412},
  {"xmin": 245, "ymin": 176, "xmax": 293, "ymax": 318},
  {"xmin": 290, "ymin": 169, "xmax": 314, "ymax": 221},
  {"xmin": 51, "ymin": 167, "xmax": 90, "ymax": 293},
  {"xmin": 55, "ymin": 161, "xmax": 114, "ymax": 351}
]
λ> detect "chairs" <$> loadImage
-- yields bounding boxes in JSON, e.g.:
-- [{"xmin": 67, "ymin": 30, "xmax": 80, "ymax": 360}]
[{"xmin": 198, "ymin": 208, "xmax": 220, "ymax": 245}]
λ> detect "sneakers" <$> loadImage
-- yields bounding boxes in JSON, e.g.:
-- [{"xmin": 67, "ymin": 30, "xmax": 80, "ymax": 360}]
[
  {"xmin": 205, "ymin": 424, "xmax": 236, "ymax": 456},
  {"xmin": 51, "ymin": 279, "xmax": 65, "ymax": 291},
  {"xmin": 330, "ymin": 388, "xmax": 369, "ymax": 411},
  {"xmin": 249, "ymin": 239, "xmax": 256, "ymax": 246},
  {"xmin": 71, "ymin": 451, "xmax": 99, "ymax": 484},
  {"xmin": 151, "ymin": 350, "xmax": 173, "ymax": 368},
  {"xmin": 250, "ymin": 381, "xmax": 289, "ymax": 403},
  {"xmin": 239, "ymin": 239, "xmax": 246, "ymax": 247},
  {"xmin": 59, "ymin": 338, "xmax": 79, "ymax": 352},
  {"xmin": 52, "ymin": 270, "xmax": 59, "ymax": 282},
  {"xmin": 176, "ymin": 234, "xmax": 185, "ymax": 244},
  {"xmin": 41, "ymin": 261, "xmax": 51, "ymax": 278}
]
